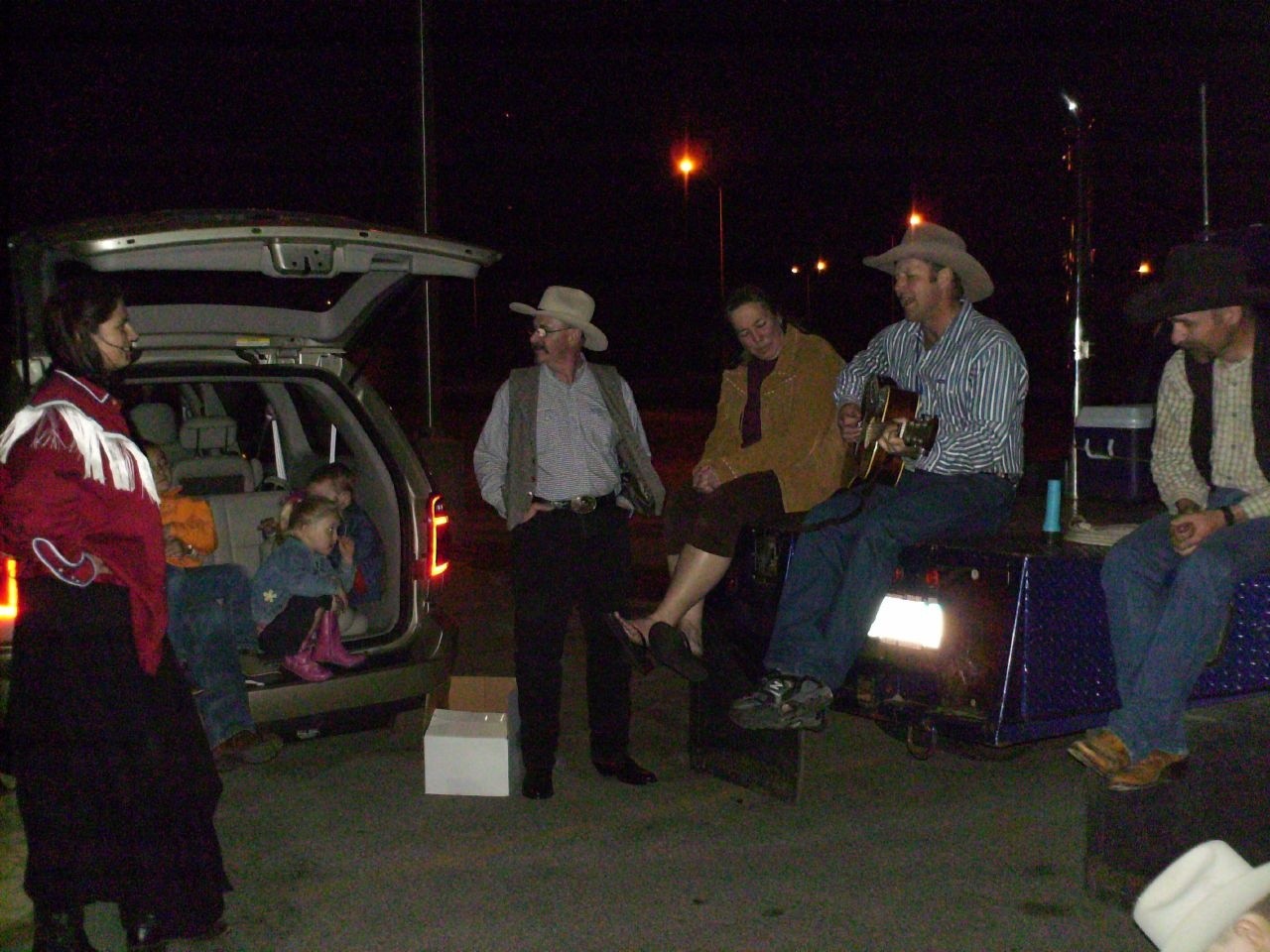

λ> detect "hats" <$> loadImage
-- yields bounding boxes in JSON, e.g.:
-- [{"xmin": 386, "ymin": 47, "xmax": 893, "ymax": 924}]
[
  {"xmin": 1118, "ymin": 235, "xmax": 1270, "ymax": 322},
  {"xmin": 509, "ymin": 286, "xmax": 609, "ymax": 351},
  {"xmin": 1130, "ymin": 839, "xmax": 1270, "ymax": 952},
  {"xmin": 863, "ymin": 221, "xmax": 995, "ymax": 304}
]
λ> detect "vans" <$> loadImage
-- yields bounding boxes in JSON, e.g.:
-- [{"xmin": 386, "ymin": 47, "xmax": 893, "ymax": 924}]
[{"xmin": 1, "ymin": 206, "xmax": 507, "ymax": 725}]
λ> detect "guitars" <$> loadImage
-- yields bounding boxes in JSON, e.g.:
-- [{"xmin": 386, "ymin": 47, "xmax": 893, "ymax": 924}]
[{"xmin": 852, "ymin": 373, "xmax": 939, "ymax": 488}]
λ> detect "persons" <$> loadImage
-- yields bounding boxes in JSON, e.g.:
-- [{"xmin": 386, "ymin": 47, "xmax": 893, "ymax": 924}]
[
  {"xmin": 1061, "ymin": 237, "xmax": 1270, "ymax": 794},
  {"xmin": 470, "ymin": 285, "xmax": 665, "ymax": 801},
  {"xmin": 249, "ymin": 492, "xmax": 366, "ymax": 681},
  {"xmin": 139, "ymin": 437, "xmax": 279, "ymax": 765},
  {"xmin": 728, "ymin": 222, "xmax": 1029, "ymax": 741},
  {"xmin": 597, "ymin": 282, "xmax": 853, "ymax": 690},
  {"xmin": 0, "ymin": 259, "xmax": 234, "ymax": 952},
  {"xmin": 306, "ymin": 459, "xmax": 389, "ymax": 606}
]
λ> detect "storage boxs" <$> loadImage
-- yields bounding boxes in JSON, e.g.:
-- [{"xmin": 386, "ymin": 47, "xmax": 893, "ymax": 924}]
[
  {"xmin": 422, "ymin": 675, "xmax": 523, "ymax": 796},
  {"xmin": 1076, "ymin": 405, "xmax": 1171, "ymax": 501}
]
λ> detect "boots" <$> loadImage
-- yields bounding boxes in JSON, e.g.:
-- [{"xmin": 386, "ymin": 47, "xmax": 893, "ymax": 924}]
[
  {"xmin": 32, "ymin": 902, "xmax": 98, "ymax": 952},
  {"xmin": 126, "ymin": 925, "xmax": 166, "ymax": 952},
  {"xmin": 282, "ymin": 632, "xmax": 333, "ymax": 682},
  {"xmin": 313, "ymin": 611, "xmax": 367, "ymax": 669}
]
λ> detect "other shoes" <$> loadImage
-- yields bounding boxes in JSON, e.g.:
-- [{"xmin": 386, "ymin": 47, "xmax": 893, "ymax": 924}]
[
  {"xmin": 1065, "ymin": 729, "xmax": 1130, "ymax": 780},
  {"xmin": 1108, "ymin": 748, "xmax": 1189, "ymax": 791}
]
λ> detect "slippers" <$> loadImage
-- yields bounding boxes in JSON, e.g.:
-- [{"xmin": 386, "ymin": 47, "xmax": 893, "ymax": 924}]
[
  {"xmin": 605, "ymin": 611, "xmax": 654, "ymax": 675},
  {"xmin": 648, "ymin": 621, "xmax": 710, "ymax": 684}
]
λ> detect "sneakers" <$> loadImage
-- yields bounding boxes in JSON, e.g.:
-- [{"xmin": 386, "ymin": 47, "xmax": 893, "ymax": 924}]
[
  {"xmin": 212, "ymin": 732, "xmax": 281, "ymax": 772},
  {"xmin": 728, "ymin": 674, "xmax": 833, "ymax": 732},
  {"xmin": 237, "ymin": 649, "xmax": 281, "ymax": 685}
]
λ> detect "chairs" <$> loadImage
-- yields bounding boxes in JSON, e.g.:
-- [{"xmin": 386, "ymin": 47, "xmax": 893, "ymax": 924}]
[{"xmin": 129, "ymin": 401, "xmax": 265, "ymax": 495}]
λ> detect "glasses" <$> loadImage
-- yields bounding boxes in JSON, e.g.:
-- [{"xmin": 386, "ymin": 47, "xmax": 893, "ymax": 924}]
[{"xmin": 526, "ymin": 325, "xmax": 569, "ymax": 337}]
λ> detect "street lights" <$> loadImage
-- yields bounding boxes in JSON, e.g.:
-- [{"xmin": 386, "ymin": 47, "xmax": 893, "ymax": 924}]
[
  {"xmin": 678, "ymin": 156, "xmax": 730, "ymax": 375},
  {"xmin": 792, "ymin": 262, "xmax": 812, "ymax": 332}
]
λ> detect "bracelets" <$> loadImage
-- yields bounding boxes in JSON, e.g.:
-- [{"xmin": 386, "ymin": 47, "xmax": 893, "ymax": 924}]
[
  {"xmin": 1220, "ymin": 506, "xmax": 1236, "ymax": 526},
  {"xmin": 176, "ymin": 539, "xmax": 187, "ymax": 554}
]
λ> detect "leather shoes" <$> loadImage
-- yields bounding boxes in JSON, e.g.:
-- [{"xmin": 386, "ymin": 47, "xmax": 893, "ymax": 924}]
[
  {"xmin": 521, "ymin": 767, "xmax": 554, "ymax": 800},
  {"xmin": 592, "ymin": 754, "xmax": 658, "ymax": 784}
]
